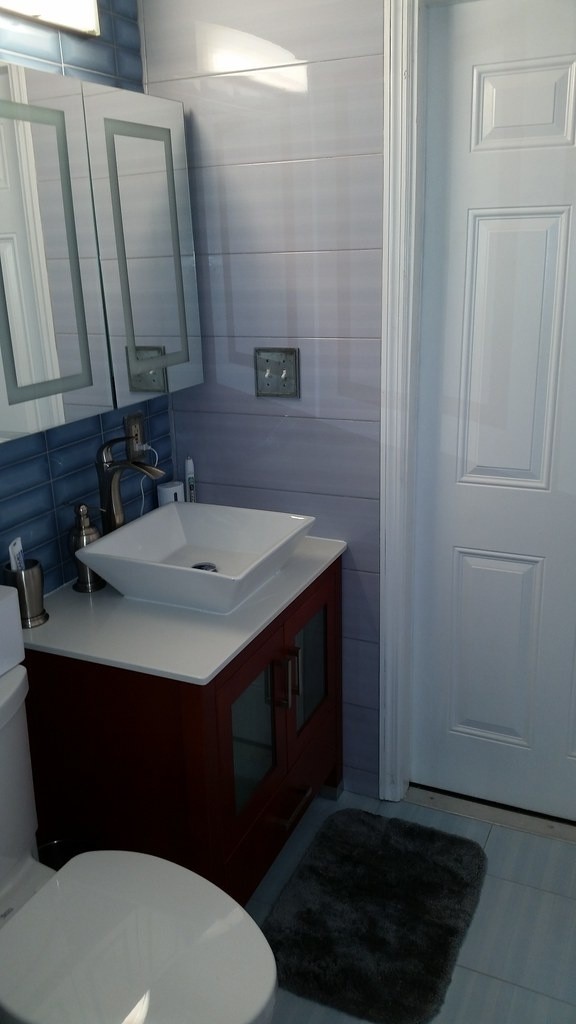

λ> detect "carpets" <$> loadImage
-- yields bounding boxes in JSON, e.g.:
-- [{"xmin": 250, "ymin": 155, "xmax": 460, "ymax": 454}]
[{"xmin": 260, "ymin": 809, "xmax": 487, "ymax": 1024}]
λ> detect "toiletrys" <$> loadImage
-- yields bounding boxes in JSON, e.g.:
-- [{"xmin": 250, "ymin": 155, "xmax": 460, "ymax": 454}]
[
  {"xmin": 67, "ymin": 503, "xmax": 109, "ymax": 592},
  {"xmin": 8, "ymin": 537, "xmax": 27, "ymax": 572}
]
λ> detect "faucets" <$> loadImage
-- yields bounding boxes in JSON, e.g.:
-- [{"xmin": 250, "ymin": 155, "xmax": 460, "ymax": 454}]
[{"xmin": 97, "ymin": 435, "xmax": 165, "ymax": 531}]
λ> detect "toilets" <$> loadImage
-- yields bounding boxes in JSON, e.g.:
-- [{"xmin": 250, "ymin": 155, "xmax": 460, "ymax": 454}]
[{"xmin": 2, "ymin": 666, "xmax": 279, "ymax": 1023}]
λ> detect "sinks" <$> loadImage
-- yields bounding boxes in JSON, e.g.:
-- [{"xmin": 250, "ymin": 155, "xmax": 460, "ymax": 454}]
[{"xmin": 75, "ymin": 498, "xmax": 317, "ymax": 611}]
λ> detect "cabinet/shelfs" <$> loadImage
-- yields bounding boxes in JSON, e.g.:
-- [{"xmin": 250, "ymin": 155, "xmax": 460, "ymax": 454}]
[
  {"xmin": 0, "ymin": 63, "xmax": 204, "ymax": 444},
  {"xmin": 21, "ymin": 534, "xmax": 346, "ymax": 908}
]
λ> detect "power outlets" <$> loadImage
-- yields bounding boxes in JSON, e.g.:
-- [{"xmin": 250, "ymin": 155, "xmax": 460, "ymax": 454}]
[{"xmin": 123, "ymin": 415, "xmax": 147, "ymax": 461}]
